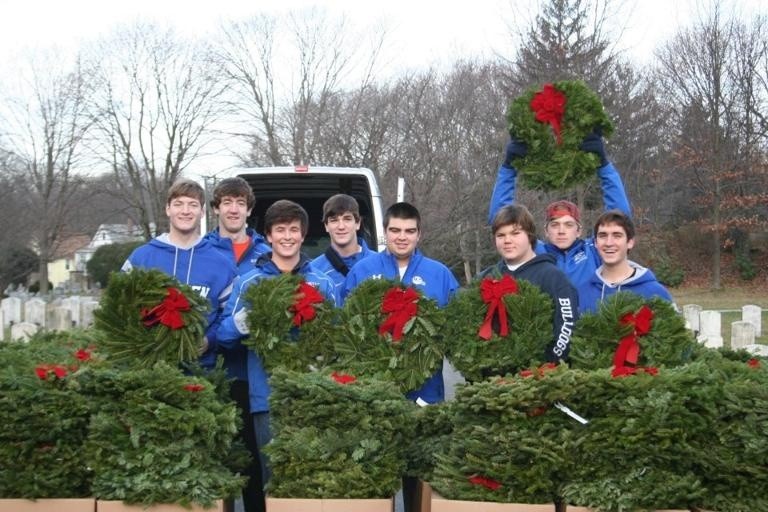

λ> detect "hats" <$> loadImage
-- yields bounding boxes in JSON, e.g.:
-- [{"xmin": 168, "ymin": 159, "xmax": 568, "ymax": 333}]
[{"xmin": 546, "ymin": 201, "xmax": 581, "ymax": 221}]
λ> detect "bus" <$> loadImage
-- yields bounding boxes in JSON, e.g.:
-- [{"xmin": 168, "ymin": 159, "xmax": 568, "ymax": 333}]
[{"xmin": 232, "ymin": 166, "xmax": 385, "ymax": 259}]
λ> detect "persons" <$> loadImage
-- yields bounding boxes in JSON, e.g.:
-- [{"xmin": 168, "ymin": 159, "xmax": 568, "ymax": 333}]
[
  {"xmin": 486, "ymin": 129, "xmax": 633, "ymax": 290},
  {"xmin": 307, "ymin": 192, "xmax": 379, "ymax": 309},
  {"xmin": 468, "ymin": 203, "xmax": 579, "ymax": 379},
  {"xmin": 120, "ymin": 177, "xmax": 240, "ymax": 383},
  {"xmin": 575, "ymin": 209, "xmax": 674, "ymax": 325},
  {"xmin": 202, "ymin": 175, "xmax": 274, "ymax": 511},
  {"xmin": 216, "ymin": 200, "xmax": 342, "ymax": 495},
  {"xmin": 337, "ymin": 202, "xmax": 465, "ymax": 512}
]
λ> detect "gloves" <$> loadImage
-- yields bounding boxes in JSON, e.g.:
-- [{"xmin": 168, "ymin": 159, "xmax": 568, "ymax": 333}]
[
  {"xmin": 198, "ymin": 336, "xmax": 208, "ymax": 352},
  {"xmin": 581, "ymin": 136, "xmax": 609, "ymax": 165},
  {"xmin": 235, "ymin": 307, "xmax": 251, "ymax": 335},
  {"xmin": 504, "ymin": 142, "xmax": 526, "ymax": 166}
]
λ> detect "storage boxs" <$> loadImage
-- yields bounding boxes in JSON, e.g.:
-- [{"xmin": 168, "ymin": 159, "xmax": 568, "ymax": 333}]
[
  {"xmin": 263, "ymin": 493, "xmax": 395, "ymax": 512},
  {"xmin": 97, "ymin": 496, "xmax": 234, "ymax": 512},
  {"xmin": 412, "ymin": 476, "xmax": 429, "ymax": 511},
  {"xmin": 429, "ymin": 489, "xmax": 556, "ymax": 512},
  {"xmin": 558, "ymin": 498, "xmax": 596, "ymax": 512},
  {"xmin": 1, "ymin": 496, "xmax": 95, "ymax": 512}
]
